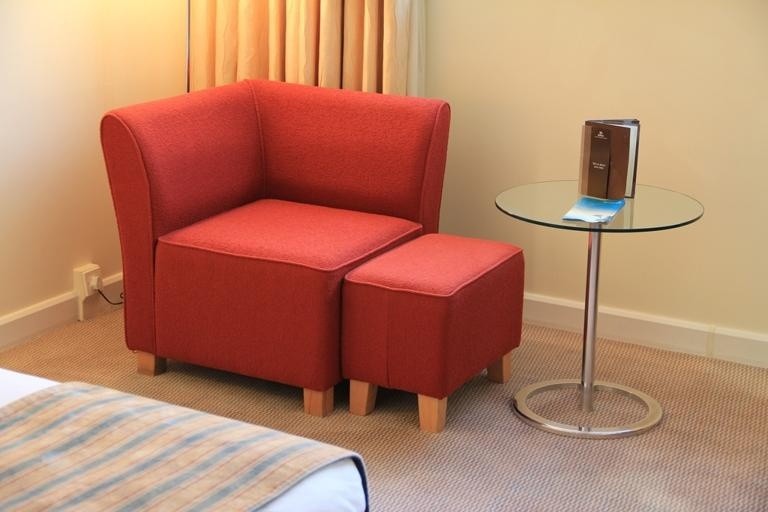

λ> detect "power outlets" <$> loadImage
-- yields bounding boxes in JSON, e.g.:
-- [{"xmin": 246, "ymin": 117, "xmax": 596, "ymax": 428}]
[{"xmin": 71, "ymin": 262, "xmax": 101, "ymax": 297}]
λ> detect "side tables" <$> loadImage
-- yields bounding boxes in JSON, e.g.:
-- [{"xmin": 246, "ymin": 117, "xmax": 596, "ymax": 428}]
[{"xmin": 495, "ymin": 180, "xmax": 704, "ymax": 438}]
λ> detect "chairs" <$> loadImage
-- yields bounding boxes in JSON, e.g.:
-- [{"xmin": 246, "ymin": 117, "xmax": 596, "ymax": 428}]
[{"xmin": 101, "ymin": 78, "xmax": 451, "ymax": 416}]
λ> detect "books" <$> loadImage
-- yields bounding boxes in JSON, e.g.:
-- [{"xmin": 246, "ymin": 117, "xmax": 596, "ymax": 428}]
[{"xmin": 561, "ymin": 119, "xmax": 640, "ymax": 223}]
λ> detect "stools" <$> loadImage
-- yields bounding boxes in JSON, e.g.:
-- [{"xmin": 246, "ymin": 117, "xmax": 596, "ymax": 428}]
[{"xmin": 340, "ymin": 233, "xmax": 525, "ymax": 432}]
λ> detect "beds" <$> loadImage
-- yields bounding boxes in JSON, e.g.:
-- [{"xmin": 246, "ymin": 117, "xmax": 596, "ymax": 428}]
[{"xmin": 2, "ymin": 367, "xmax": 368, "ymax": 512}]
